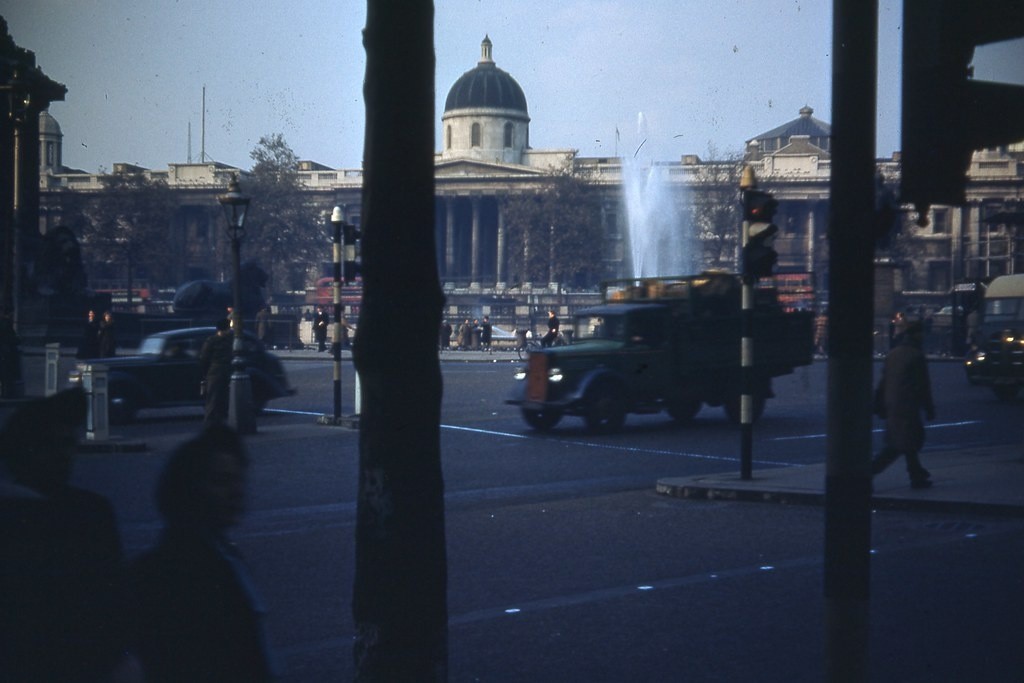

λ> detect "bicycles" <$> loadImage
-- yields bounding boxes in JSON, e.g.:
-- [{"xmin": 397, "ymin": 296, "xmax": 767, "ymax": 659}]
[{"xmin": 517, "ymin": 334, "xmax": 566, "ymax": 362}]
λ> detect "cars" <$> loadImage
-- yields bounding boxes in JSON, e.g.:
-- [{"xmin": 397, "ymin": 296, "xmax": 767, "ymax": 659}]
[{"xmin": 70, "ymin": 327, "xmax": 299, "ymax": 428}]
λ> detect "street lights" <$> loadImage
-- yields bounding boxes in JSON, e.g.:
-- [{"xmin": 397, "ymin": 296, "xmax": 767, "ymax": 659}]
[
  {"xmin": 216, "ymin": 171, "xmax": 254, "ymax": 436},
  {"xmin": 0, "ymin": 62, "xmax": 43, "ymax": 339}
]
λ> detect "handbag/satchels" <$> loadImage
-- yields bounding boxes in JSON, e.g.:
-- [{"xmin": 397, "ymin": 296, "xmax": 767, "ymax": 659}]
[{"xmin": 874, "ymin": 387, "xmax": 886, "ymax": 418}]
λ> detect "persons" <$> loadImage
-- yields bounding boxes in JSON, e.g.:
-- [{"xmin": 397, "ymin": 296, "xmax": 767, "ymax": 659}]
[
  {"xmin": 122, "ymin": 426, "xmax": 273, "ymax": 683},
  {"xmin": 195, "ymin": 320, "xmax": 234, "ymax": 429},
  {"xmin": 458, "ymin": 315, "xmax": 493, "ymax": 350},
  {"xmin": 966, "ymin": 304, "xmax": 979, "ymax": 349},
  {"xmin": 77, "ymin": 308, "xmax": 119, "ymax": 359},
  {"xmin": 540, "ymin": 311, "xmax": 559, "ymax": 348},
  {"xmin": 0, "ymin": 385, "xmax": 126, "ymax": 683},
  {"xmin": 254, "ymin": 304, "xmax": 273, "ymax": 343},
  {"xmin": 873, "ymin": 321, "xmax": 934, "ymax": 488},
  {"xmin": 169, "ymin": 344, "xmax": 189, "ymax": 357},
  {"xmin": 313, "ymin": 305, "xmax": 329, "ymax": 352},
  {"xmin": 0, "ymin": 305, "xmax": 22, "ymax": 393},
  {"xmin": 441, "ymin": 320, "xmax": 452, "ymax": 349},
  {"xmin": 892, "ymin": 312, "xmax": 907, "ymax": 338},
  {"xmin": 341, "ymin": 313, "xmax": 356, "ymax": 346}
]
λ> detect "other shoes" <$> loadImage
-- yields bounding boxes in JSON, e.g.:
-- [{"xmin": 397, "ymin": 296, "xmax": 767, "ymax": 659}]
[{"xmin": 911, "ymin": 480, "xmax": 930, "ymax": 489}]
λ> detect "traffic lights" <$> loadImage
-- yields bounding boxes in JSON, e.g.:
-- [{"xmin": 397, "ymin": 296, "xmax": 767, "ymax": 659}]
[
  {"xmin": 742, "ymin": 190, "xmax": 781, "ymax": 285},
  {"xmin": 341, "ymin": 224, "xmax": 363, "ymax": 283}
]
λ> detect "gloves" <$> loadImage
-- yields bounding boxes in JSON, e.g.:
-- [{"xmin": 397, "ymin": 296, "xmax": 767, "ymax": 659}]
[{"xmin": 926, "ymin": 407, "xmax": 936, "ymax": 419}]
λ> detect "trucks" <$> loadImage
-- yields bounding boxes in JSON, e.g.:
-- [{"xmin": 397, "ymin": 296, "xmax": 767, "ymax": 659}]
[
  {"xmin": 501, "ymin": 278, "xmax": 816, "ymax": 435},
  {"xmin": 965, "ymin": 273, "xmax": 1023, "ymax": 384}
]
